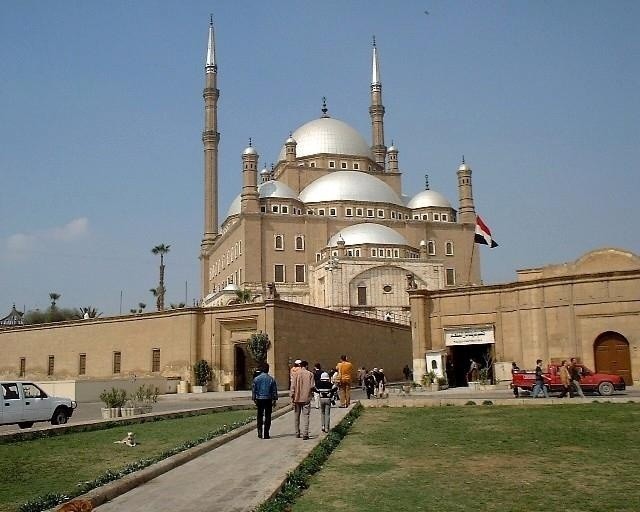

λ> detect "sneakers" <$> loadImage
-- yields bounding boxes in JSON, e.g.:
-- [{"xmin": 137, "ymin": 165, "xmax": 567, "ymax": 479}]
[{"xmin": 322, "ymin": 426, "xmax": 328, "ymax": 433}]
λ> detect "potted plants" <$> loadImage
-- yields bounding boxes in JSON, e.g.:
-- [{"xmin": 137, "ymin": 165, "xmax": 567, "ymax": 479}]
[
  {"xmin": 190, "ymin": 359, "xmax": 213, "ymax": 394},
  {"xmin": 421, "ymin": 370, "xmax": 436, "ymax": 392},
  {"xmin": 99, "ymin": 385, "xmax": 159, "ymax": 417}
]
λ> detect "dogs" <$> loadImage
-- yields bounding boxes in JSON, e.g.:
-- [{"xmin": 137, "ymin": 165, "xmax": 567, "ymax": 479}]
[{"xmin": 113, "ymin": 432, "xmax": 136, "ymax": 448}]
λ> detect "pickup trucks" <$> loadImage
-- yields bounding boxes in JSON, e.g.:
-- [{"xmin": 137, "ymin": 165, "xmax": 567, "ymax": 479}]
[{"xmin": 509, "ymin": 358, "xmax": 625, "ymax": 397}]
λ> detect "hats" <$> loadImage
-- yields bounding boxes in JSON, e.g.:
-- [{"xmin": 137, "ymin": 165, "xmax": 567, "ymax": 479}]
[
  {"xmin": 321, "ymin": 372, "xmax": 330, "ymax": 380},
  {"xmin": 294, "ymin": 359, "xmax": 302, "ymax": 365}
]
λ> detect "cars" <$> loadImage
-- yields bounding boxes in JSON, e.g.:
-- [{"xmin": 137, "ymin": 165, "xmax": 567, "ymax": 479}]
[{"xmin": 0, "ymin": 380, "xmax": 77, "ymax": 429}]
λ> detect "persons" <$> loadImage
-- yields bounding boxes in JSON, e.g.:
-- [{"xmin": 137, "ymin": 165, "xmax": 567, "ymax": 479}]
[
  {"xmin": 290, "ymin": 361, "xmax": 316, "ymax": 440},
  {"xmin": 445, "ymin": 356, "xmax": 477, "ymax": 388},
  {"xmin": 403, "ymin": 364, "xmax": 409, "ymax": 381},
  {"xmin": 335, "ymin": 355, "xmax": 353, "ymax": 407},
  {"xmin": 251, "ymin": 363, "xmax": 278, "ymax": 439},
  {"xmin": 511, "ymin": 358, "xmax": 586, "ymax": 398},
  {"xmin": 315, "ymin": 372, "xmax": 337, "ymax": 433},
  {"xmin": 288, "ymin": 359, "xmax": 386, "ymax": 408}
]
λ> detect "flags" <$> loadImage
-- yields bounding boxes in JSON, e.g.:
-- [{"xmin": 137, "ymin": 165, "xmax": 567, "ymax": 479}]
[{"xmin": 474, "ymin": 215, "xmax": 499, "ymax": 249}]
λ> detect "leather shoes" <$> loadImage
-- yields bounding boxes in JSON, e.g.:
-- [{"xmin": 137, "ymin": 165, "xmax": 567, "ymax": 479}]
[{"xmin": 296, "ymin": 434, "xmax": 313, "ymax": 440}]
